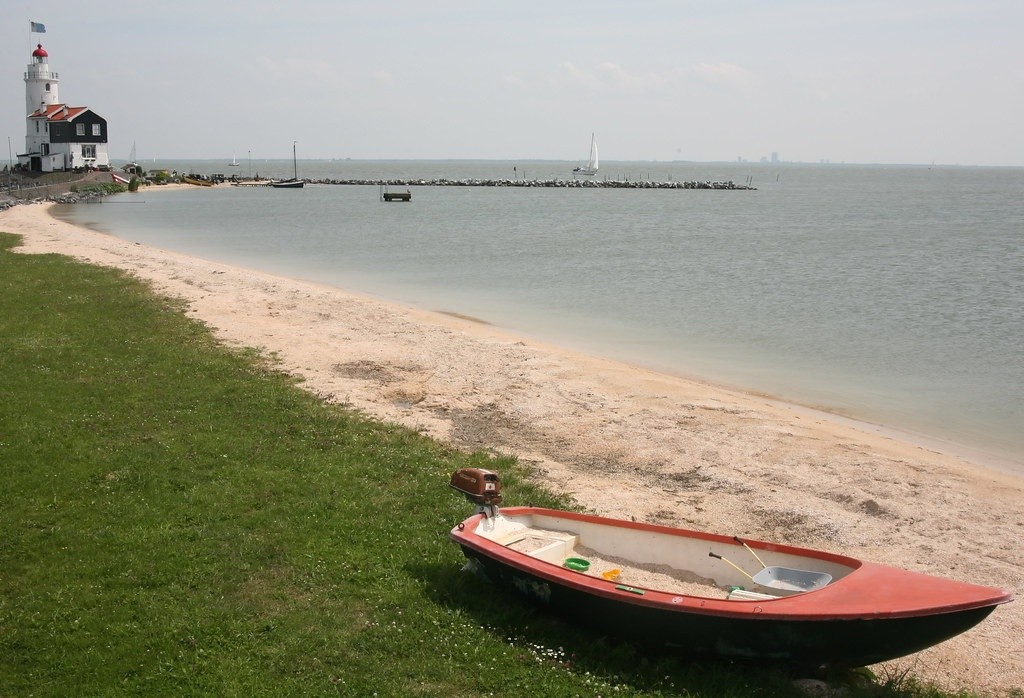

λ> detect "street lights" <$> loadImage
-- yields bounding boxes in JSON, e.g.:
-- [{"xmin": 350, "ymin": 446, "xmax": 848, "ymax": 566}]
[{"xmin": 7, "ymin": 135, "xmax": 13, "ymax": 169}]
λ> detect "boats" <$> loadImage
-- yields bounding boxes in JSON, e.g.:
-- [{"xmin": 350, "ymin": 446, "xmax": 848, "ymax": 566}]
[
  {"xmin": 448, "ymin": 467, "xmax": 1017, "ymax": 670},
  {"xmin": 272, "ymin": 181, "xmax": 305, "ymax": 188},
  {"xmin": 185, "ymin": 176, "xmax": 213, "ymax": 186},
  {"xmin": 111, "ymin": 174, "xmax": 129, "ymax": 183}
]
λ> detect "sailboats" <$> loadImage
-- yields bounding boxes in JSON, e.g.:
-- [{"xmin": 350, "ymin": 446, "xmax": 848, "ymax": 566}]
[
  {"xmin": 571, "ymin": 133, "xmax": 600, "ymax": 175},
  {"xmin": 229, "ymin": 156, "xmax": 239, "ymax": 166}
]
